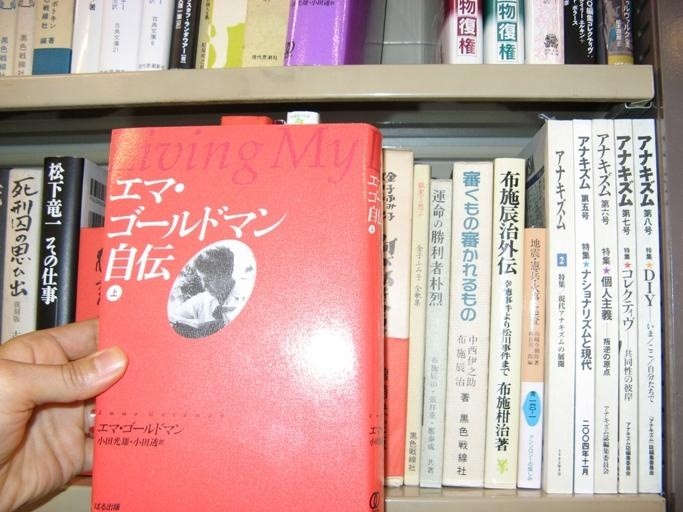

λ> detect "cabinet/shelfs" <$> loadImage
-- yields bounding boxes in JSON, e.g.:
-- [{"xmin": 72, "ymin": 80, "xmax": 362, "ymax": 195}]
[{"xmin": 0, "ymin": 0, "xmax": 683, "ymax": 512}]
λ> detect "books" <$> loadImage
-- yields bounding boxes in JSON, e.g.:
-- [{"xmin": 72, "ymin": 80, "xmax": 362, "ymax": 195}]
[
  {"xmin": 1, "ymin": 0, "xmax": 636, "ymax": 75},
  {"xmin": 0, "ymin": 111, "xmax": 661, "ymax": 511}
]
